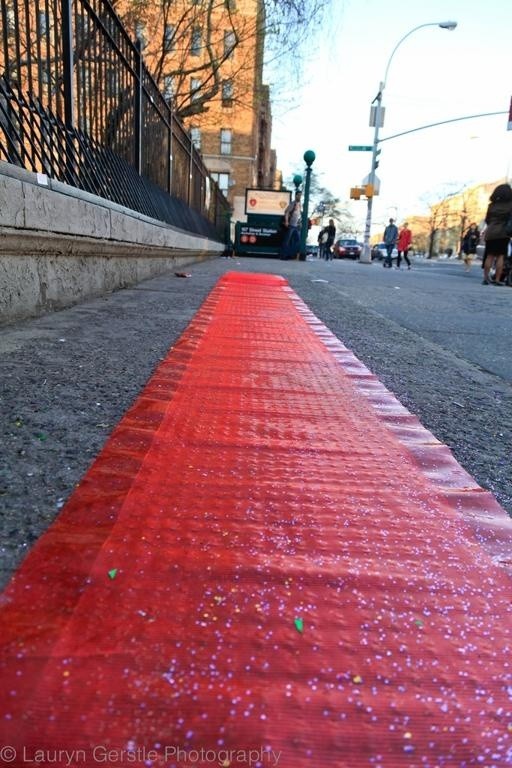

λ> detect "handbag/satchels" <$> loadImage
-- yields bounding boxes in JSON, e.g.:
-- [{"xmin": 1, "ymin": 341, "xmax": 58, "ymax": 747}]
[{"xmin": 281, "ymin": 216, "xmax": 290, "ymax": 229}]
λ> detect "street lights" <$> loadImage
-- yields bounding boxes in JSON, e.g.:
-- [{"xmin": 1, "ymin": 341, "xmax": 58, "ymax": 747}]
[
  {"xmin": 360, "ymin": 20, "xmax": 458, "ymax": 261},
  {"xmin": 298, "ymin": 150, "xmax": 316, "ymax": 261},
  {"xmin": 293, "ymin": 174, "xmax": 303, "ymax": 201}
]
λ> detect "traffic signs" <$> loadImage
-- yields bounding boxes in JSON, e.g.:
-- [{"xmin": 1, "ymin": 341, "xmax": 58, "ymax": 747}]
[{"xmin": 349, "ymin": 145, "xmax": 373, "ymax": 150}]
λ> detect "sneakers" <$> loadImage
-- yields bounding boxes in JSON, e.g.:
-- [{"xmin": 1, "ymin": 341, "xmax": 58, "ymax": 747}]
[{"xmin": 483, "ymin": 278, "xmax": 506, "ymax": 286}]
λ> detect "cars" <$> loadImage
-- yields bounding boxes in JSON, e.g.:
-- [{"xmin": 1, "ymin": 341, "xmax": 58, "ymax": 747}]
[
  {"xmin": 371, "ymin": 242, "xmax": 404, "ymax": 260},
  {"xmin": 475, "ymin": 219, "xmax": 512, "ymax": 260},
  {"xmin": 334, "ymin": 239, "xmax": 360, "ymax": 259}
]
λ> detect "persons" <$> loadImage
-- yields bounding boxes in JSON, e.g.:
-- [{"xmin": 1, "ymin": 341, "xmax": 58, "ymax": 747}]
[
  {"xmin": 280, "ymin": 191, "xmax": 302, "ymax": 260},
  {"xmin": 462, "ymin": 223, "xmax": 479, "ymax": 272},
  {"xmin": 317, "ymin": 219, "xmax": 336, "ymax": 260},
  {"xmin": 382, "ymin": 218, "xmax": 398, "ymax": 267},
  {"xmin": 395, "ymin": 222, "xmax": 412, "ymax": 270},
  {"xmin": 481, "ymin": 183, "xmax": 512, "ymax": 286}
]
